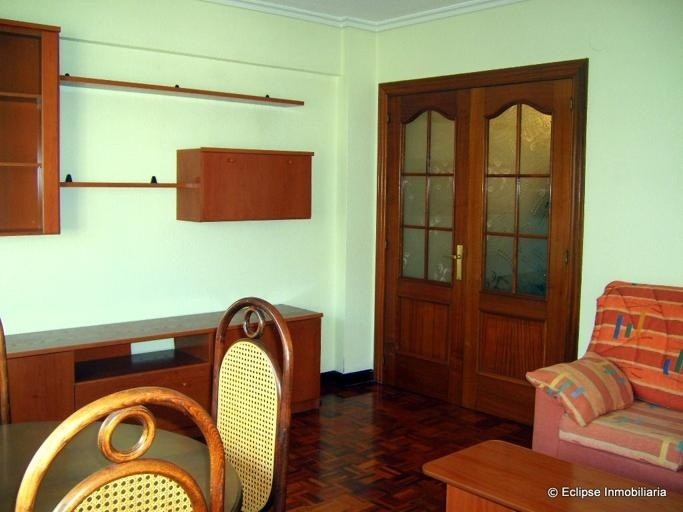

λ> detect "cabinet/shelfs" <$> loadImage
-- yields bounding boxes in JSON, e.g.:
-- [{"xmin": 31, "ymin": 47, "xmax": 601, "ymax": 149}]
[
  {"xmin": 0, "ymin": 302, "xmax": 324, "ymax": 438},
  {"xmin": 0, "ymin": 19, "xmax": 61, "ymax": 236},
  {"xmin": 57, "ymin": 71, "xmax": 315, "ymax": 223}
]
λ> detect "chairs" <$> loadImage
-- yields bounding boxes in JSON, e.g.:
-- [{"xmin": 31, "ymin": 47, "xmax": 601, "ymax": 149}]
[
  {"xmin": 533, "ymin": 280, "xmax": 682, "ymax": 496},
  {"xmin": 14, "ymin": 385, "xmax": 227, "ymax": 512},
  {"xmin": 212, "ymin": 298, "xmax": 297, "ymax": 512}
]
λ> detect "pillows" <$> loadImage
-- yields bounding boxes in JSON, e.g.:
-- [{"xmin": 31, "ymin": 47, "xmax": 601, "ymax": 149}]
[{"xmin": 525, "ymin": 352, "xmax": 635, "ymax": 427}]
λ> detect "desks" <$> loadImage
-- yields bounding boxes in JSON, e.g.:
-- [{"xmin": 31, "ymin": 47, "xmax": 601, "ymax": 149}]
[
  {"xmin": 421, "ymin": 440, "xmax": 683, "ymax": 512},
  {"xmin": 1, "ymin": 405, "xmax": 242, "ymax": 512}
]
